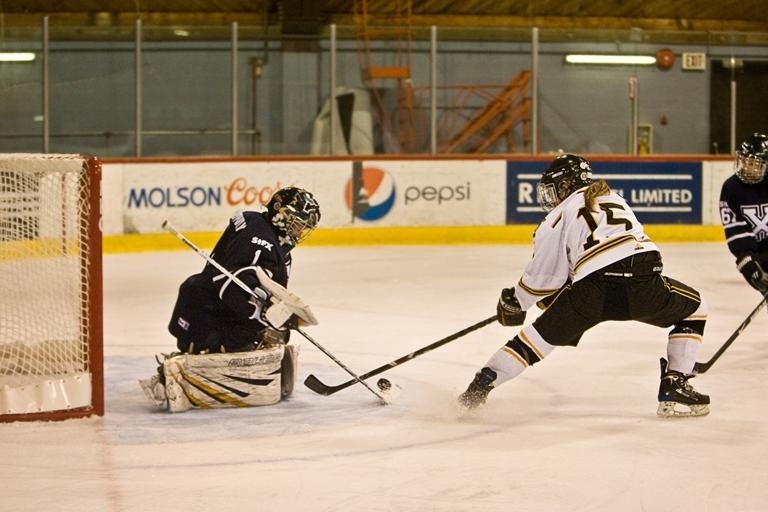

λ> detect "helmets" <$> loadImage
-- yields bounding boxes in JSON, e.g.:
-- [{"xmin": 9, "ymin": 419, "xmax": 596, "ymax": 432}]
[
  {"xmin": 536, "ymin": 154, "xmax": 593, "ymax": 214},
  {"xmin": 733, "ymin": 132, "xmax": 768, "ymax": 186},
  {"xmin": 267, "ymin": 186, "xmax": 322, "ymax": 248}
]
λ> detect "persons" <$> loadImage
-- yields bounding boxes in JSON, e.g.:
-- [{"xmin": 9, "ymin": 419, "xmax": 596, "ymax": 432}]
[
  {"xmin": 719, "ymin": 131, "xmax": 768, "ymax": 301},
  {"xmin": 460, "ymin": 152, "xmax": 710, "ymax": 404},
  {"xmin": 147, "ymin": 187, "xmax": 321, "ymax": 404}
]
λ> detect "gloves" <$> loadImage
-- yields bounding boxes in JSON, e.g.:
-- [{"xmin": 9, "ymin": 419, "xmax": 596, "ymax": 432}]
[
  {"xmin": 733, "ymin": 253, "xmax": 768, "ymax": 296},
  {"xmin": 496, "ymin": 286, "xmax": 528, "ymax": 329}
]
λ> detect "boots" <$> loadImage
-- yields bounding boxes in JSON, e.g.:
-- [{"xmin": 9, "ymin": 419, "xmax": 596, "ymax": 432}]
[
  {"xmin": 456, "ymin": 370, "xmax": 497, "ymax": 409},
  {"xmin": 655, "ymin": 355, "xmax": 712, "ymax": 406}
]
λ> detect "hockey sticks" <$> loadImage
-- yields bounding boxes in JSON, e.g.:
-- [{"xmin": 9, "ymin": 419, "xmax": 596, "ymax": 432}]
[
  {"xmin": 303, "ymin": 316, "xmax": 497, "ymax": 395},
  {"xmin": 691, "ymin": 292, "xmax": 768, "ymax": 374},
  {"xmin": 161, "ymin": 221, "xmax": 403, "ymax": 404}
]
